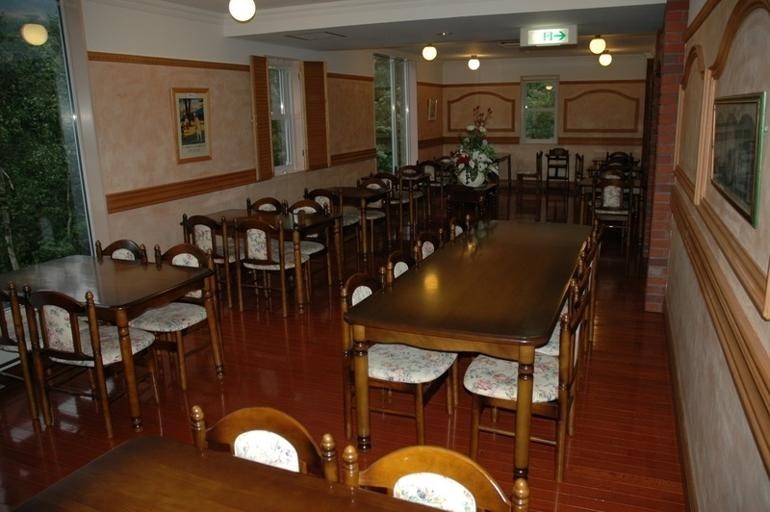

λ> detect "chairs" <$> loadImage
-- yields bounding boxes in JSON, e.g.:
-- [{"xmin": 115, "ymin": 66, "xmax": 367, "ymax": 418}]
[
  {"xmin": 517, "ymin": 147, "xmax": 641, "ymax": 246},
  {"xmin": 23, "ymin": 284, "xmax": 160, "ymax": 441},
  {"xmin": 192, "ymin": 404, "xmax": 336, "ymax": 480},
  {"xmin": 128, "ymin": 243, "xmax": 223, "ymax": 390},
  {"xmin": 0, "ymin": 281, "xmax": 38, "ymax": 420},
  {"xmin": 96, "ymin": 240, "xmax": 147, "ymax": 263},
  {"xmin": 344, "ymin": 441, "xmax": 530, "ymax": 512}
]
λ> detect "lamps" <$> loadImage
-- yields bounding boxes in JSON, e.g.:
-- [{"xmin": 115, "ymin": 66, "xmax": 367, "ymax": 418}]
[
  {"xmin": 21, "ymin": 14, "xmax": 49, "ymax": 45},
  {"xmin": 589, "ymin": 34, "xmax": 607, "ymax": 54},
  {"xmin": 468, "ymin": 55, "xmax": 480, "ymax": 70},
  {"xmin": 599, "ymin": 50, "xmax": 612, "ymax": 67},
  {"xmin": 229, "ymin": 0, "xmax": 256, "ymax": 22},
  {"xmin": 423, "ymin": 44, "xmax": 437, "ymax": 61}
]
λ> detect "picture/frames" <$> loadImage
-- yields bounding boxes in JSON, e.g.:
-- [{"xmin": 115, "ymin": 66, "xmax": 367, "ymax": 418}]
[
  {"xmin": 710, "ymin": 91, "xmax": 767, "ymax": 229},
  {"xmin": 428, "ymin": 97, "xmax": 438, "ymax": 122},
  {"xmin": 170, "ymin": 87, "xmax": 213, "ymax": 165}
]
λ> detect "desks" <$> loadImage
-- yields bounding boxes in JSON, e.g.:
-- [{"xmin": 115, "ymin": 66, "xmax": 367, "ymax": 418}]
[
  {"xmin": 9, "ymin": 433, "xmax": 450, "ymax": 512},
  {"xmin": 1, "ymin": 253, "xmax": 224, "ymax": 435}
]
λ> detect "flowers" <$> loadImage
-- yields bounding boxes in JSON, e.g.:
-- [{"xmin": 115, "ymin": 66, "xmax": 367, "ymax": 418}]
[{"xmin": 445, "ymin": 104, "xmax": 501, "ymax": 184}]
